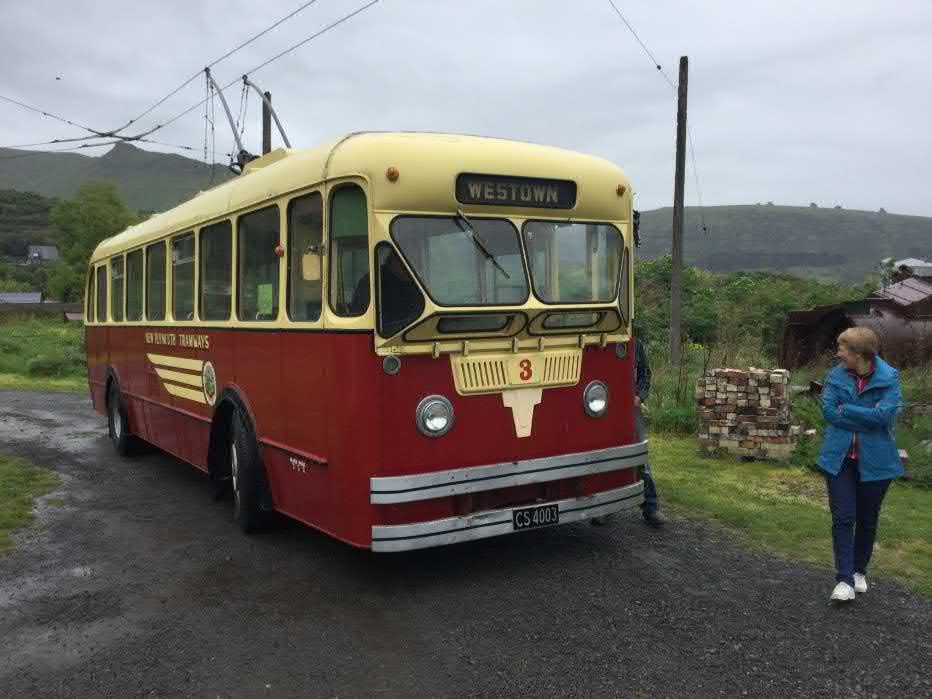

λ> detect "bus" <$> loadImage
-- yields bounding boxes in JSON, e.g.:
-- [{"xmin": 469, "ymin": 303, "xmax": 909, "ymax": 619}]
[{"xmin": 84, "ymin": 67, "xmax": 649, "ymax": 553}]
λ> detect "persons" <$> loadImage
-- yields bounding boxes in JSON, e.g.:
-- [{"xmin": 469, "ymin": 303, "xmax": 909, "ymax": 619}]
[
  {"xmin": 352, "ymin": 239, "xmax": 421, "ymax": 322},
  {"xmin": 814, "ymin": 326, "xmax": 906, "ymax": 601},
  {"xmin": 631, "ymin": 333, "xmax": 670, "ymax": 527}
]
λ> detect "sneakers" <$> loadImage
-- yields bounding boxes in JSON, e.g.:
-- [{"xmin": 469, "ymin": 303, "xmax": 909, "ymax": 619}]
[
  {"xmin": 852, "ymin": 571, "xmax": 868, "ymax": 593},
  {"xmin": 829, "ymin": 580, "xmax": 856, "ymax": 602}
]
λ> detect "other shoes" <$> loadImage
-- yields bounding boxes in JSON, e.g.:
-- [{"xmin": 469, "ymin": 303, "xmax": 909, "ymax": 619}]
[
  {"xmin": 591, "ymin": 515, "xmax": 610, "ymax": 528},
  {"xmin": 642, "ymin": 509, "xmax": 666, "ymax": 528}
]
